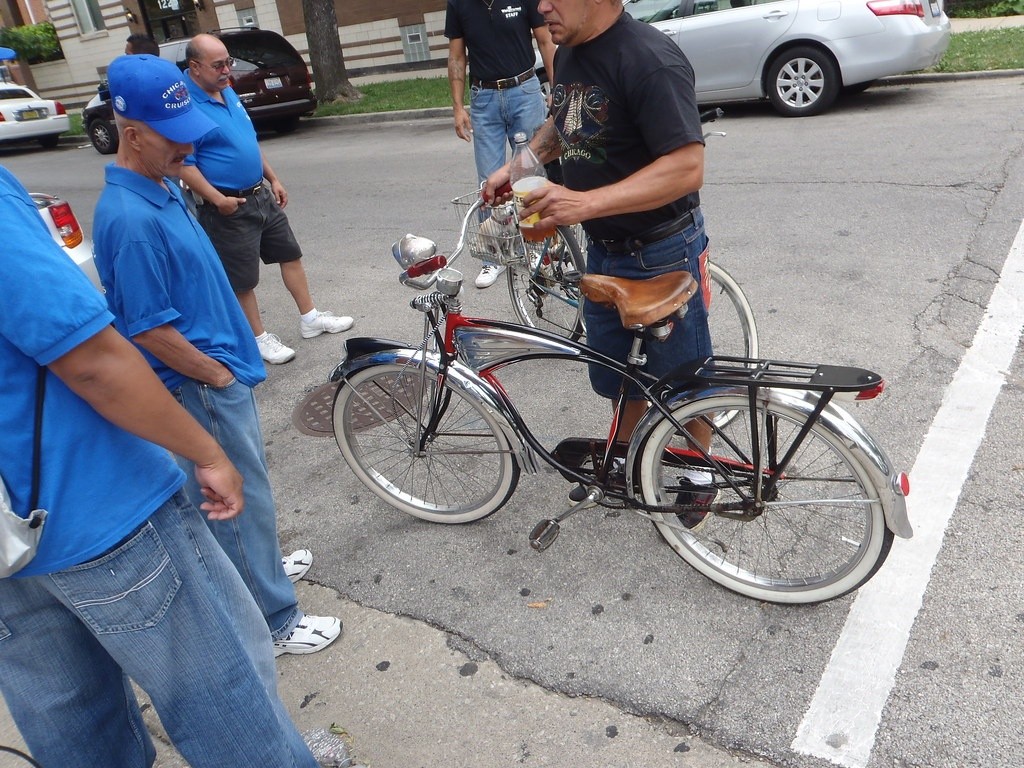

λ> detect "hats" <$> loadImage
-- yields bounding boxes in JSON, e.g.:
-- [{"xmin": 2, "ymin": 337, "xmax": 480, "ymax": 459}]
[{"xmin": 108, "ymin": 55, "xmax": 222, "ymax": 144}]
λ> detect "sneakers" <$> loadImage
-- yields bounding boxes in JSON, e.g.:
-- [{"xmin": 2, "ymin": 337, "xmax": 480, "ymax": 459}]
[
  {"xmin": 272, "ymin": 614, "xmax": 343, "ymax": 659},
  {"xmin": 300, "ymin": 311, "xmax": 354, "ymax": 338},
  {"xmin": 475, "ymin": 263, "xmax": 507, "ymax": 288},
  {"xmin": 281, "ymin": 549, "xmax": 313, "ymax": 582},
  {"xmin": 258, "ymin": 333, "xmax": 296, "ymax": 365},
  {"xmin": 671, "ymin": 473, "xmax": 721, "ymax": 534},
  {"xmin": 530, "ymin": 248, "xmax": 576, "ymax": 277},
  {"xmin": 568, "ymin": 485, "xmax": 599, "ymax": 508}
]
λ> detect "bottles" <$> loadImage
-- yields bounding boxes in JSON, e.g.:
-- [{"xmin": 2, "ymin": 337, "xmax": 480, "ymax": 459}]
[{"xmin": 509, "ymin": 132, "xmax": 557, "ymax": 241}]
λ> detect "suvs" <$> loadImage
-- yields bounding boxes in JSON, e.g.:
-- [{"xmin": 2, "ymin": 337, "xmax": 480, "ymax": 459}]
[{"xmin": 80, "ymin": 26, "xmax": 319, "ymax": 155}]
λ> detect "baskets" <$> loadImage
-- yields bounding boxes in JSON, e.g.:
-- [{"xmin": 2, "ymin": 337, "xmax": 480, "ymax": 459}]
[{"xmin": 451, "ymin": 180, "xmax": 557, "ymax": 264}]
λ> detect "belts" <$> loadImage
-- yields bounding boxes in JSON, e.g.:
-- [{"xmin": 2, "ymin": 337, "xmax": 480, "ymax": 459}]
[
  {"xmin": 472, "ymin": 68, "xmax": 537, "ymax": 89},
  {"xmin": 219, "ymin": 177, "xmax": 264, "ymax": 197},
  {"xmin": 590, "ymin": 206, "xmax": 700, "ymax": 257}
]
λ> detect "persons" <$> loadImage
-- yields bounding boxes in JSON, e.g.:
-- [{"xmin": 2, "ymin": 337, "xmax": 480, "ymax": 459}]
[
  {"xmin": 0, "ymin": 166, "xmax": 318, "ymax": 768},
  {"xmin": 178, "ymin": 33, "xmax": 350, "ymax": 368},
  {"xmin": 443, "ymin": 0, "xmax": 557, "ymax": 288},
  {"xmin": 124, "ymin": 33, "xmax": 160, "ymax": 57},
  {"xmin": 480, "ymin": 0, "xmax": 721, "ymax": 535},
  {"xmin": 90, "ymin": 53, "xmax": 343, "ymax": 658}
]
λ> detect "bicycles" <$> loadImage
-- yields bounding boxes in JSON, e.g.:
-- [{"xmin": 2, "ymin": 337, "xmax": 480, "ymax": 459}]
[
  {"xmin": 449, "ymin": 179, "xmax": 759, "ymax": 436},
  {"xmin": 327, "ymin": 178, "xmax": 915, "ymax": 606}
]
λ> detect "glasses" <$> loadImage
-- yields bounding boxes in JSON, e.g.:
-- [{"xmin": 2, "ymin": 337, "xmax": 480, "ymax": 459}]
[{"xmin": 190, "ymin": 56, "xmax": 236, "ymax": 72}]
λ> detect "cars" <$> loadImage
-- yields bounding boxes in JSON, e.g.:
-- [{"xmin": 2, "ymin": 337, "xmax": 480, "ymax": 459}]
[
  {"xmin": 533, "ymin": 0, "xmax": 952, "ymax": 118},
  {"xmin": 0, "ymin": 81, "xmax": 71, "ymax": 148},
  {"xmin": 28, "ymin": 191, "xmax": 107, "ymax": 296}
]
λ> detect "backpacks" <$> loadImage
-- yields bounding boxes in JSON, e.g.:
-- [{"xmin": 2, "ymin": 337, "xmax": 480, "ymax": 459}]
[{"xmin": 2, "ymin": 366, "xmax": 54, "ymax": 576}]
